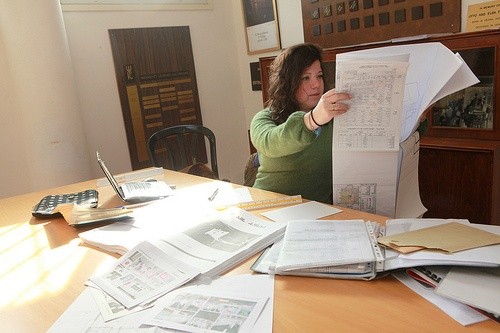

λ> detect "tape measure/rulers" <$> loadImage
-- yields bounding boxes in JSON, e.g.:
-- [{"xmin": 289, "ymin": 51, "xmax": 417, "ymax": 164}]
[{"xmin": 217, "ymin": 195, "xmax": 302, "ymax": 210}]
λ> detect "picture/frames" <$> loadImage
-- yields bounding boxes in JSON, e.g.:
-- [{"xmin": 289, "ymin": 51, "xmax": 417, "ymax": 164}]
[{"xmin": 240, "ymin": 0, "xmax": 282, "ymax": 55}]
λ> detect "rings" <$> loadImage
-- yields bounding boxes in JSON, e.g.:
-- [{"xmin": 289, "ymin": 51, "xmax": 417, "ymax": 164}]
[{"xmin": 331, "ymin": 103, "xmax": 336, "ymax": 110}]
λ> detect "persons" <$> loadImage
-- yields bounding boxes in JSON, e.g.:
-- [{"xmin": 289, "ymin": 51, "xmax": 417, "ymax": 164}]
[{"xmin": 249, "ymin": 43, "xmax": 352, "ymax": 204}]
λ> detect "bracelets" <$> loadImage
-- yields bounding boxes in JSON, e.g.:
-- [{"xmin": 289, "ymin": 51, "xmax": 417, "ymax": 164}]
[
  {"xmin": 308, "ymin": 112, "xmax": 320, "ymax": 135},
  {"xmin": 311, "ymin": 110, "xmax": 323, "ymax": 127}
]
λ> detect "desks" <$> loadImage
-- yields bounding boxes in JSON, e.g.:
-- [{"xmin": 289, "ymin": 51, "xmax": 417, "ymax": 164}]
[{"xmin": 0, "ymin": 167, "xmax": 500, "ymax": 333}]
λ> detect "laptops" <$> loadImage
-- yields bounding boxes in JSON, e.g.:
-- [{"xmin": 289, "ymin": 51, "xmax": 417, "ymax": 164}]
[{"xmin": 96, "ymin": 152, "xmax": 174, "ymax": 203}]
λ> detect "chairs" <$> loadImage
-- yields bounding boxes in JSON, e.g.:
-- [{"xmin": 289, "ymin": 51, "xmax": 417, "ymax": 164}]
[{"xmin": 147, "ymin": 125, "xmax": 219, "ymax": 180}]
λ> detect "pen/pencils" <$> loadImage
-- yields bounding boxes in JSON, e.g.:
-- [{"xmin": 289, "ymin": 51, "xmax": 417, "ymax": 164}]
[{"xmin": 208, "ymin": 188, "xmax": 219, "ymax": 200}]
